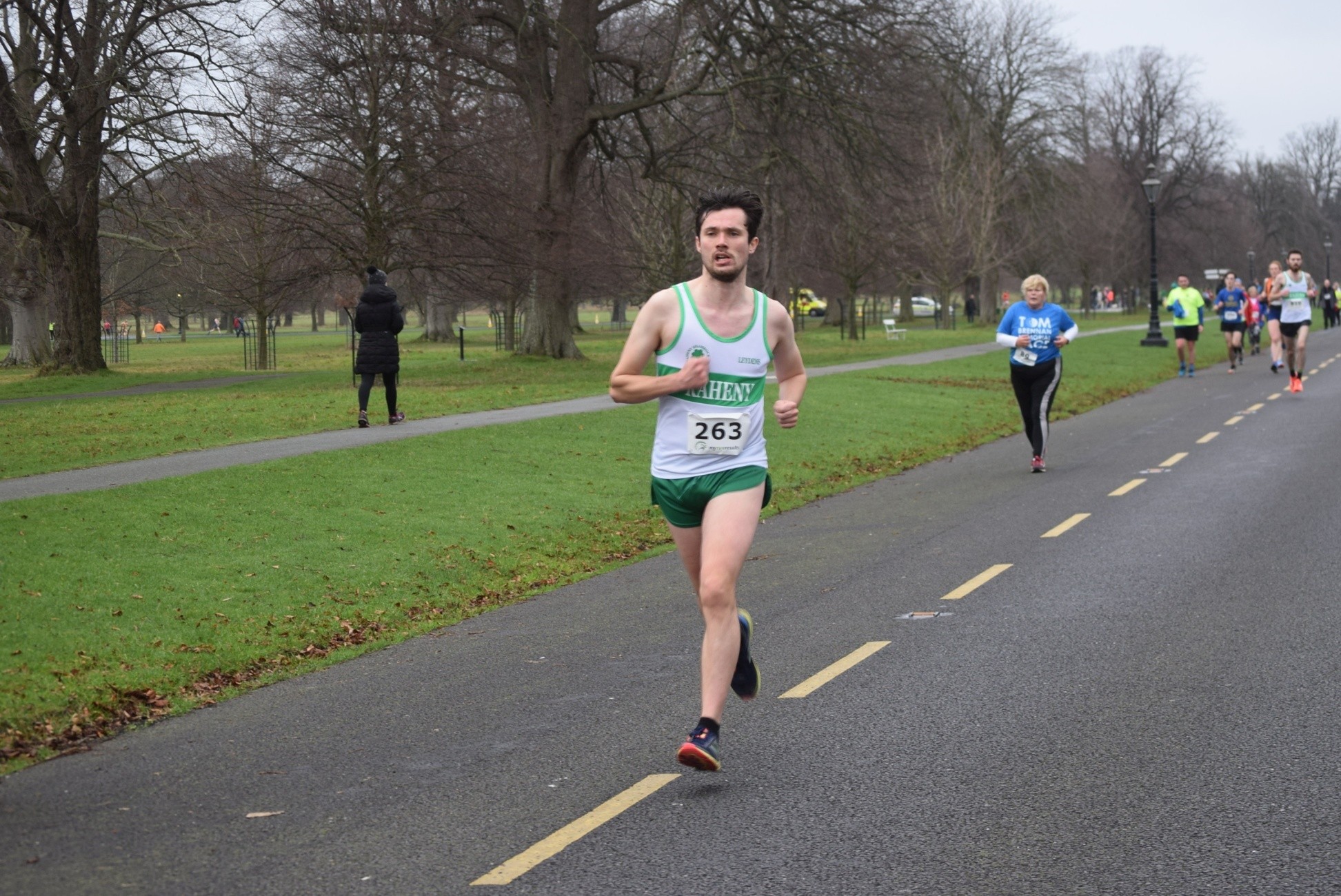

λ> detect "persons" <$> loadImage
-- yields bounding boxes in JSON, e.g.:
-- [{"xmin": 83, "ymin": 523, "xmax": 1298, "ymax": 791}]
[
  {"xmin": 355, "ymin": 266, "xmax": 405, "ymax": 428},
  {"xmin": 104, "ymin": 319, "xmax": 111, "ymax": 336},
  {"xmin": 120, "ymin": 319, "xmax": 128, "ymax": 336},
  {"xmin": 207, "ymin": 315, "xmax": 223, "ymax": 335},
  {"xmin": 49, "ymin": 320, "xmax": 55, "ymax": 340},
  {"xmin": 154, "ymin": 320, "xmax": 166, "ymax": 342},
  {"xmin": 1201, "ymin": 286, "xmax": 1215, "ymax": 308},
  {"xmin": 1310, "ymin": 278, "xmax": 1341, "ymax": 330},
  {"xmin": 234, "ymin": 316, "xmax": 244, "ymax": 335},
  {"xmin": 965, "ymin": 294, "xmax": 977, "ymax": 323},
  {"xmin": 1211, "ymin": 260, "xmax": 1289, "ymax": 374},
  {"xmin": 996, "ymin": 274, "xmax": 1078, "ymax": 473},
  {"xmin": 1089, "ymin": 284, "xmax": 1136, "ymax": 309},
  {"xmin": 1003, "ymin": 287, "xmax": 1011, "ymax": 309},
  {"xmin": 1268, "ymin": 249, "xmax": 1318, "ymax": 394},
  {"xmin": 1166, "ymin": 274, "xmax": 1205, "ymax": 377},
  {"xmin": 608, "ymin": 189, "xmax": 807, "ymax": 771}
]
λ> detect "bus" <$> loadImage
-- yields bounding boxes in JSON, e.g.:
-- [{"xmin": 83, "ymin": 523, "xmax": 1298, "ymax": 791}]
[{"xmin": 789, "ymin": 288, "xmax": 827, "ymax": 317}]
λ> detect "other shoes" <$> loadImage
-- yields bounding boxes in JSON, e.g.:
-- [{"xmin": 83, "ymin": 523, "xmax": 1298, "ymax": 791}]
[
  {"xmin": 1278, "ymin": 361, "xmax": 1283, "ymax": 368},
  {"xmin": 1239, "ymin": 358, "xmax": 1242, "ymax": 364},
  {"xmin": 1271, "ymin": 364, "xmax": 1278, "ymax": 374},
  {"xmin": 1228, "ymin": 365, "xmax": 1237, "ymax": 374},
  {"xmin": 1256, "ymin": 347, "xmax": 1259, "ymax": 353},
  {"xmin": 1290, "ymin": 372, "xmax": 1297, "ymax": 393},
  {"xmin": 1188, "ymin": 369, "xmax": 1194, "ymax": 377},
  {"xmin": 1294, "ymin": 379, "xmax": 1303, "ymax": 391},
  {"xmin": 1178, "ymin": 367, "xmax": 1186, "ymax": 377},
  {"xmin": 1251, "ymin": 350, "xmax": 1254, "ymax": 355}
]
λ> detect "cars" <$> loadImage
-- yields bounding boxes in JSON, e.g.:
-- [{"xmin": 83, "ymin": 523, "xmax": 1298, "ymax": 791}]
[{"xmin": 893, "ymin": 297, "xmax": 954, "ymax": 318}]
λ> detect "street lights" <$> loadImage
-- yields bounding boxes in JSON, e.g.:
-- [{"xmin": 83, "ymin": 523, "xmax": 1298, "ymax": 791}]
[
  {"xmin": 1323, "ymin": 235, "xmax": 1334, "ymax": 278},
  {"xmin": 1141, "ymin": 162, "xmax": 1169, "ymax": 347},
  {"xmin": 1246, "ymin": 246, "xmax": 1257, "ymax": 283}
]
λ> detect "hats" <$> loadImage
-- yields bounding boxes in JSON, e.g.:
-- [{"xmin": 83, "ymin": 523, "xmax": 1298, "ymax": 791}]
[{"xmin": 367, "ymin": 265, "xmax": 387, "ymax": 285}]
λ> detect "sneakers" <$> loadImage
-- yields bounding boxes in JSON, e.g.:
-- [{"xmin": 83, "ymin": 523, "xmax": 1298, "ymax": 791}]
[
  {"xmin": 358, "ymin": 410, "xmax": 370, "ymax": 427},
  {"xmin": 388, "ymin": 412, "xmax": 406, "ymax": 425},
  {"xmin": 730, "ymin": 608, "xmax": 761, "ymax": 701},
  {"xmin": 1031, "ymin": 456, "xmax": 1046, "ymax": 473},
  {"xmin": 677, "ymin": 724, "xmax": 721, "ymax": 771}
]
